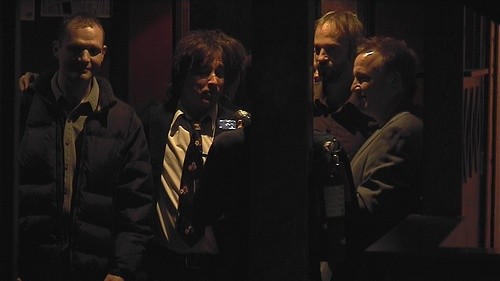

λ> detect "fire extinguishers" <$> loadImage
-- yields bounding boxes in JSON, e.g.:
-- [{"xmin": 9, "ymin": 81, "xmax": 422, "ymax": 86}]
[{"xmin": 320, "ymin": 147, "xmax": 359, "ymax": 249}]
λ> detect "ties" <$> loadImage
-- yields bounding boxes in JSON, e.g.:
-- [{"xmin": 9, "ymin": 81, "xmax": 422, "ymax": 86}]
[{"xmin": 175, "ymin": 123, "xmax": 205, "ymax": 249}]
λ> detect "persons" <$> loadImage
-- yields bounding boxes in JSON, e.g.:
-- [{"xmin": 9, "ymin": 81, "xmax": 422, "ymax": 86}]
[
  {"xmin": 20, "ymin": 12, "xmax": 158, "ymax": 281},
  {"xmin": 21, "ymin": 30, "xmax": 261, "ymax": 281},
  {"xmin": 313, "ymin": 11, "xmax": 393, "ymax": 281},
  {"xmin": 329, "ymin": 38, "xmax": 424, "ymax": 281}
]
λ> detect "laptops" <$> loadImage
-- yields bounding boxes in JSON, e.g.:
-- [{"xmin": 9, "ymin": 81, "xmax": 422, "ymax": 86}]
[{"xmin": 365, "ymin": 214, "xmax": 484, "ymax": 256}]
